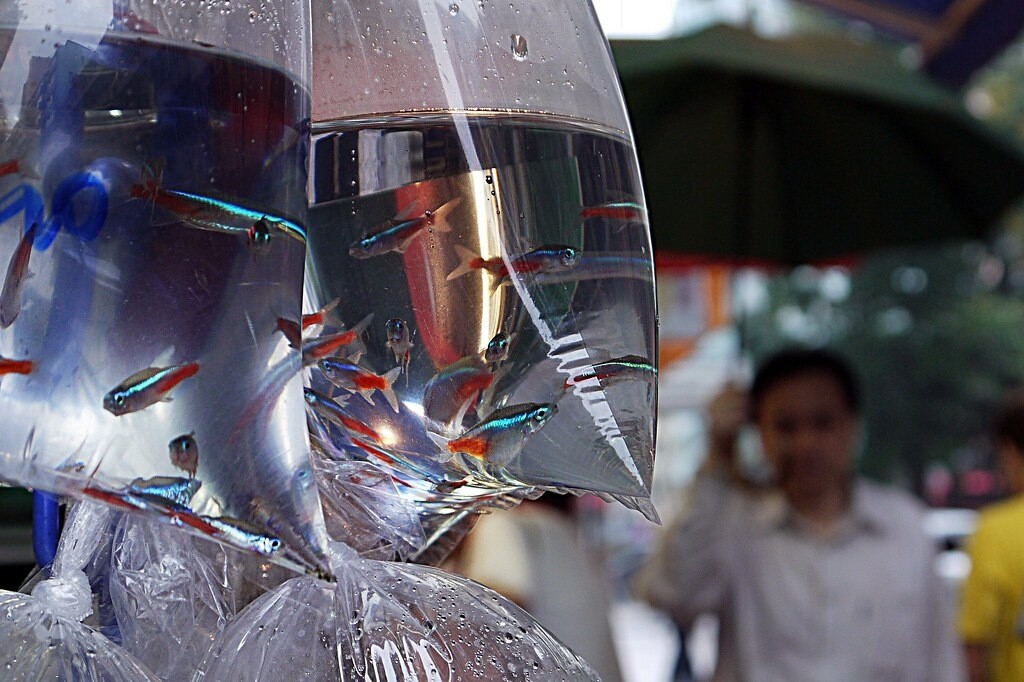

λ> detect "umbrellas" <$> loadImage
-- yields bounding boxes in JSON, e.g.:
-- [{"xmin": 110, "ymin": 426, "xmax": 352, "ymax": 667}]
[{"xmin": 607, "ymin": 23, "xmax": 1022, "ymax": 381}]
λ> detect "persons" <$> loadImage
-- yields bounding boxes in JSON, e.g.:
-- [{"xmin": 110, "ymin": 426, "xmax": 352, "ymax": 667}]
[
  {"xmin": 465, "ymin": 490, "xmax": 623, "ymax": 682},
  {"xmin": 647, "ymin": 341, "xmax": 968, "ymax": 682},
  {"xmin": 955, "ymin": 415, "xmax": 1024, "ymax": 682}
]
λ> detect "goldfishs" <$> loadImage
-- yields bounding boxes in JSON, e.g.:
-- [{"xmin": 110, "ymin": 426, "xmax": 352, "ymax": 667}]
[{"xmin": 0, "ymin": 148, "xmax": 659, "ymax": 579}]
